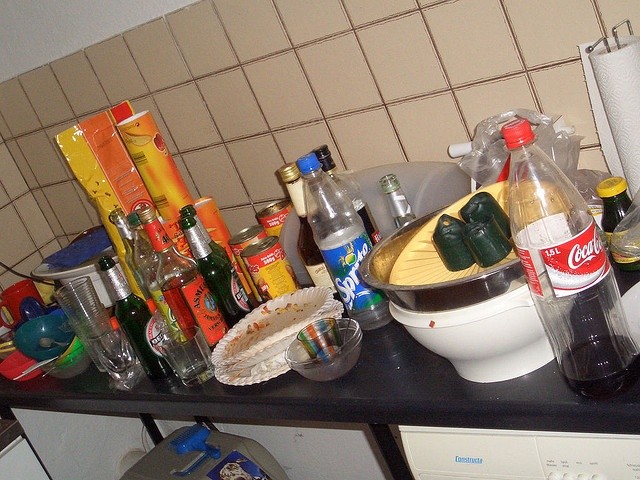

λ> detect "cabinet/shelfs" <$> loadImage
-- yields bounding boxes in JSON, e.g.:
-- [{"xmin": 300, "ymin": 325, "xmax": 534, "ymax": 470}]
[{"xmin": 0, "ymin": 409, "xmax": 640, "ymax": 480}]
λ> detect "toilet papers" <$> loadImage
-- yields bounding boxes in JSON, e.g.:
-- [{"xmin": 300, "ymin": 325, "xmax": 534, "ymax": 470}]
[{"xmin": 577, "ymin": 35, "xmax": 640, "ymax": 201}]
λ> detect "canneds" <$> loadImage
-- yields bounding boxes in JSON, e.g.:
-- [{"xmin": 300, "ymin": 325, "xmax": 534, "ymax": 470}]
[
  {"xmin": 227, "ymin": 225, "xmax": 268, "ymax": 304},
  {"xmin": 240, "ymin": 235, "xmax": 302, "ymax": 306},
  {"xmin": 255, "ymin": 198, "xmax": 294, "ymax": 238}
]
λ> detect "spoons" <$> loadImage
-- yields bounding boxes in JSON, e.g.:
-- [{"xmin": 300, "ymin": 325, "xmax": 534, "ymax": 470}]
[{"xmin": 40, "ymin": 337, "xmax": 71, "ymax": 349}]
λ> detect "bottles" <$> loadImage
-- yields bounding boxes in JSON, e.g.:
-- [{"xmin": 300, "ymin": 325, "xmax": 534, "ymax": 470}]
[
  {"xmin": 178, "ymin": 217, "xmax": 254, "ymax": 327},
  {"xmin": 127, "ymin": 209, "xmax": 191, "ymax": 343},
  {"xmin": 312, "ymin": 145, "xmax": 384, "ymax": 247},
  {"xmin": 596, "ymin": 176, "xmax": 639, "ymax": 281},
  {"xmin": 296, "ymin": 152, "xmax": 394, "ymax": 331},
  {"xmin": 137, "ymin": 206, "xmax": 235, "ymax": 351},
  {"xmin": 179, "ymin": 204, "xmax": 255, "ymax": 311},
  {"xmin": 501, "ymin": 118, "xmax": 639, "ymax": 400},
  {"xmin": 109, "ymin": 208, "xmax": 152, "ymax": 301},
  {"xmin": 98, "ymin": 254, "xmax": 178, "ymax": 380},
  {"xmin": 277, "ymin": 161, "xmax": 350, "ymax": 318},
  {"xmin": 378, "ymin": 172, "xmax": 416, "ymax": 229}
]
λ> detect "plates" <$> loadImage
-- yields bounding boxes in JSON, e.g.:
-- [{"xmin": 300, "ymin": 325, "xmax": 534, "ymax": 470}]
[
  {"xmin": 213, "ymin": 299, "xmax": 344, "ymax": 372},
  {"xmin": 30, "ymin": 226, "xmax": 121, "ymax": 281},
  {"xmin": 211, "ymin": 287, "xmax": 334, "ymax": 367},
  {"xmin": 215, "ymin": 315, "xmax": 342, "ymax": 386}
]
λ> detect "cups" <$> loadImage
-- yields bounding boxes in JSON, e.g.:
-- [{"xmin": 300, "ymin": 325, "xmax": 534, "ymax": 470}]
[
  {"xmin": 73, "ymin": 305, "xmax": 117, "ymax": 372},
  {"xmin": 0, "ymin": 278, "xmax": 43, "ymax": 329},
  {"xmin": 162, "ymin": 325, "xmax": 214, "ymax": 386},
  {"xmin": 297, "ymin": 318, "xmax": 348, "ymax": 374},
  {"xmin": 86, "ymin": 327, "xmax": 144, "ymax": 391},
  {"xmin": 54, "ymin": 276, "xmax": 98, "ymax": 325}
]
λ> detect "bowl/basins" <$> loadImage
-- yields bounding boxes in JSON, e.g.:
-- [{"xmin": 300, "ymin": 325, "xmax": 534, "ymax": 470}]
[
  {"xmin": 0, "ymin": 350, "xmax": 43, "ymax": 382},
  {"xmin": 386, "ymin": 283, "xmax": 579, "ymax": 385},
  {"xmin": 360, "ymin": 189, "xmax": 528, "ymax": 310},
  {"xmin": 15, "ymin": 312, "xmax": 73, "ymax": 360},
  {"xmin": 39, "ymin": 345, "xmax": 92, "ymax": 379},
  {"xmin": 285, "ymin": 318, "xmax": 363, "ymax": 383}
]
